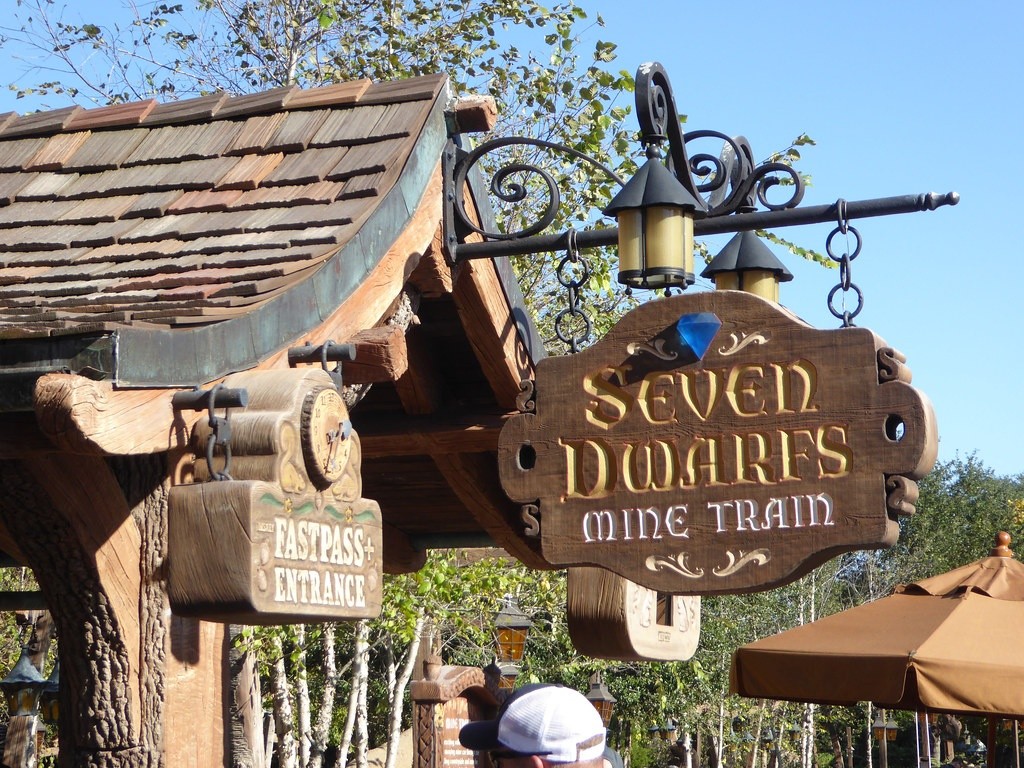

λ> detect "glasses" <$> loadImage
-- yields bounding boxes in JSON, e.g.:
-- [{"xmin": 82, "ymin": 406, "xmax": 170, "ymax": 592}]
[{"xmin": 487, "ymin": 751, "xmax": 553, "ymax": 768}]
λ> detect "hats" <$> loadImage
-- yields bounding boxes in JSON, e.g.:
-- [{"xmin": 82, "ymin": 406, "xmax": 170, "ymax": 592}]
[{"xmin": 458, "ymin": 683, "xmax": 606, "ymax": 761}]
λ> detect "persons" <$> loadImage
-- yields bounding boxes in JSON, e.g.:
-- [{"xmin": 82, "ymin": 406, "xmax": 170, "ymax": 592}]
[{"xmin": 457, "ymin": 682, "xmax": 608, "ymax": 768}]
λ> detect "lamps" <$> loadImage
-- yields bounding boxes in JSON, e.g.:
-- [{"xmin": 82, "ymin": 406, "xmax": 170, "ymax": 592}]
[
  {"xmin": 441, "ymin": 60, "xmax": 806, "ymax": 303},
  {"xmin": 0, "ymin": 624, "xmax": 59, "ymax": 724}
]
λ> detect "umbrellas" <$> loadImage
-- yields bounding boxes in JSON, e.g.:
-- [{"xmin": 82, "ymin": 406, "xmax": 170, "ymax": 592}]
[{"xmin": 729, "ymin": 531, "xmax": 1024, "ymax": 717}]
